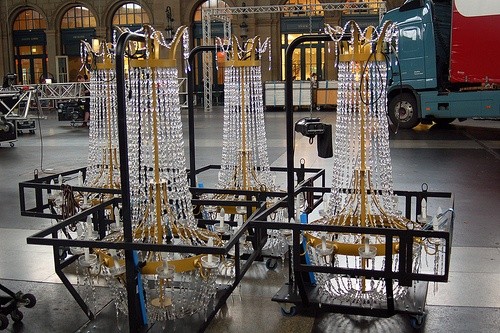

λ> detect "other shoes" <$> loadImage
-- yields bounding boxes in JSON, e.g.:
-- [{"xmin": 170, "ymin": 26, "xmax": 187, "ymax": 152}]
[{"xmin": 82, "ymin": 122, "xmax": 87, "ymax": 128}]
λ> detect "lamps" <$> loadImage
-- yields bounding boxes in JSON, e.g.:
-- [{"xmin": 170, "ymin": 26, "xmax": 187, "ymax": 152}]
[
  {"xmin": 48, "ymin": 38, "xmax": 117, "ymax": 223},
  {"xmin": 195, "ymin": 37, "xmax": 306, "ymax": 256},
  {"xmin": 69, "ymin": 25, "xmax": 252, "ymax": 330},
  {"xmin": 262, "ymin": 19, "xmax": 444, "ymax": 309}
]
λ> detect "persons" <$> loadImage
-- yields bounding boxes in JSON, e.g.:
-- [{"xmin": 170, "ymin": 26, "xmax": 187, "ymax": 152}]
[
  {"xmin": 311, "ymin": 72, "xmax": 317, "ymax": 82},
  {"xmin": 39, "ymin": 72, "xmax": 90, "ymax": 128}
]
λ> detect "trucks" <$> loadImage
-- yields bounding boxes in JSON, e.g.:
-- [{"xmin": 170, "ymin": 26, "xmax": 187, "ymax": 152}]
[{"xmin": 372, "ymin": 0, "xmax": 500, "ymax": 130}]
